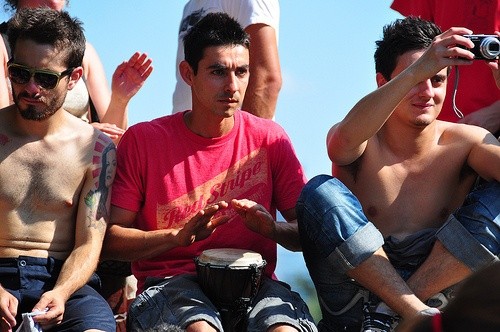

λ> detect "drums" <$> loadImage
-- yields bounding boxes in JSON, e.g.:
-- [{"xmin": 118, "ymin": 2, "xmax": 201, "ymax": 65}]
[{"xmin": 192, "ymin": 248, "xmax": 268, "ymax": 332}]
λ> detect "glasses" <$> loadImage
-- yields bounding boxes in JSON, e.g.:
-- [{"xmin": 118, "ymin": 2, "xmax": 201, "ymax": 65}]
[{"xmin": 6, "ymin": 57, "xmax": 76, "ymax": 92}]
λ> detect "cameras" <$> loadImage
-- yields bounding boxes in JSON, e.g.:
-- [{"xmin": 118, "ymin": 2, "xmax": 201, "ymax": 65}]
[{"xmin": 455, "ymin": 33, "xmax": 500, "ymax": 60}]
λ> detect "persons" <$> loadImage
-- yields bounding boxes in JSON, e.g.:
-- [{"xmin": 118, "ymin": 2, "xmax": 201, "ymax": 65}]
[
  {"xmin": 173, "ymin": 0, "xmax": 282, "ymax": 124},
  {"xmin": 0, "ymin": 6, "xmax": 119, "ymax": 332},
  {"xmin": 103, "ymin": 11, "xmax": 316, "ymax": 332},
  {"xmin": 297, "ymin": 0, "xmax": 500, "ymax": 332},
  {"xmin": 0, "ymin": 0, "xmax": 154, "ymax": 314}
]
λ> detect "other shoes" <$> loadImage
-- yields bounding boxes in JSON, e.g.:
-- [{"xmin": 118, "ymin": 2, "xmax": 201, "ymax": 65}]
[{"xmin": 362, "ymin": 302, "xmax": 396, "ymax": 332}]
